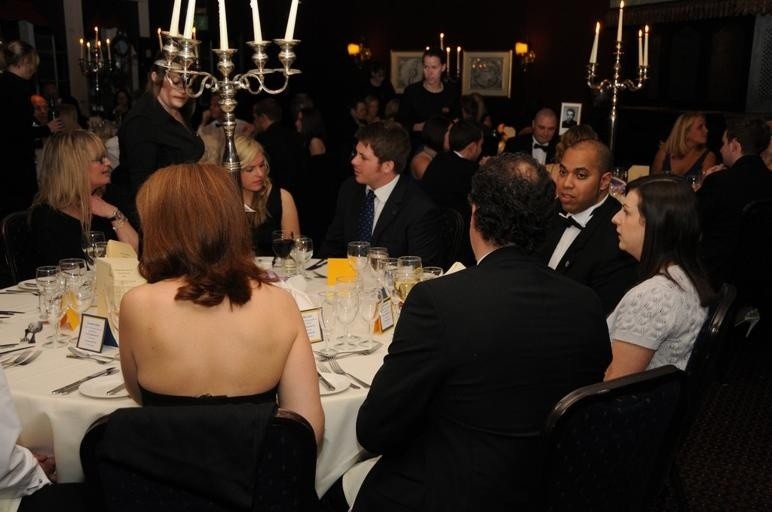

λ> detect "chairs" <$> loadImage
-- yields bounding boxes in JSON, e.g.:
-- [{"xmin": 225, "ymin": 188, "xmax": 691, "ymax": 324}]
[
  {"xmin": 538, "ymin": 363, "xmax": 685, "ymax": 511},
  {"xmin": 78, "ymin": 397, "xmax": 322, "ymax": 509},
  {"xmin": 0, "ymin": 210, "xmax": 31, "ymax": 285},
  {"xmin": 681, "ymin": 284, "xmax": 736, "ymax": 432}
]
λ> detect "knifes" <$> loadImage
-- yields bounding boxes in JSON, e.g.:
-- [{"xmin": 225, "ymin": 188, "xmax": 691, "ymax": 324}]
[{"xmin": 50, "ymin": 346, "xmax": 130, "ymax": 399}]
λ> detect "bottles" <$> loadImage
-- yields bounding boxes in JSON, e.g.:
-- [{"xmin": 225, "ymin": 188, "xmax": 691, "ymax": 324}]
[
  {"xmin": 47, "ymin": 95, "xmax": 60, "ymax": 121},
  {"xmin": 608, "ymin": 167, "xmax": 626, "ymax": 197},
  {"xmin": 84, "ymin": 102, "xmax": 122, "ymax": 126}
]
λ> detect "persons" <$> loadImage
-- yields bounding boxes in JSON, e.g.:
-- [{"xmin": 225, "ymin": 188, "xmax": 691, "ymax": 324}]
[{"xmin": 1, "ymin": 39, "xmax": 770, "ymax": 510}]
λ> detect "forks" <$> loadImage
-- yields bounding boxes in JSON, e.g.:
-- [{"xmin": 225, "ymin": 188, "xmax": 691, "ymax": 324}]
[
  {"xmin": 311, "ymin": 349, "xmax": 371, "ymax": 395},
  {"xmin": 0, "ymin": 280, "xmax": 52, "ymax": 368}
]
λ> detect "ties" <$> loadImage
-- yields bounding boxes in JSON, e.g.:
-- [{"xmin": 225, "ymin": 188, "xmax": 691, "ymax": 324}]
[{"xmin": 355, "ymin": 190, "xmax": 374, "ymax": 240}]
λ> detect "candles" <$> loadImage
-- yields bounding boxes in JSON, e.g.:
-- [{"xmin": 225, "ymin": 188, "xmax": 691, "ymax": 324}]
[
  {"xmin": 588, "ymin": 2, "xmax": 653, "ymax": 69},
  {"xmin": 169, "ymin": 1, "xmax": 301, "ymax": 52},
  {"xmin": 80, "ymin": 25, "xmax": 115, "ymax": 63},
  {"xmin": 437, "ymin": 32, "xmax": 462, "ymax": 73}
]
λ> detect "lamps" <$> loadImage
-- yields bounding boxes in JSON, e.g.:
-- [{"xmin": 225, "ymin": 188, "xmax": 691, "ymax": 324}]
[
  {"xmin": 514, "ymin": 42, "xmax": 536, "ymax": 67},
  {"xmin": 346, "ymin": 43, "xmax": 371, "ymax": 68}
]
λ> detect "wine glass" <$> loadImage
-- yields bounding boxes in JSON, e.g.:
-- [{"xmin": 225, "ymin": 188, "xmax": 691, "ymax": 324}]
[
  {"xmin": 345, "ymin": 239, "xmax": 444, "ymax": 316},
  {"xmin": 34, "ymin": 230, "xmax": 108, "ymax": 349},
  {"xmin": 272, "ymin": 229, "xmax": 314, "ymax": 279},
  {"xmin": 314, "ymin": 275, "xmax": 385, "ymax": 353}
]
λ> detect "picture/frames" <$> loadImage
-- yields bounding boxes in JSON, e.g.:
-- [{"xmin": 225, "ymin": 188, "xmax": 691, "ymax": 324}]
[
  {"xmin": 558, "ymin": 101, "xmax": 581, "ymax": 138},
  {"xmin": 390, "ymin": 50, "xmax": 425, "ymax": 94},
  {"xmin": 459, "ymin": 50, "xmax": 512, "ymax": 100}
]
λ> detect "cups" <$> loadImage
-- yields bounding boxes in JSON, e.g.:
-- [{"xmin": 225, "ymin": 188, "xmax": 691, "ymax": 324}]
[{"xmin": 687, "ymin": 175, "xmax": 700, "ymax": 189}]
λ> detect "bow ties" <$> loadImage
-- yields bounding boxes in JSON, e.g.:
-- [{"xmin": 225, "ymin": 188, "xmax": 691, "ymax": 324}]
[
  {"xmin": 534, "ymin": 144, "xmax": 549, "ymax": 152},
  {"xmin": 558, "ymin": 215, "xmax": 583, "ymax": 229}
]
